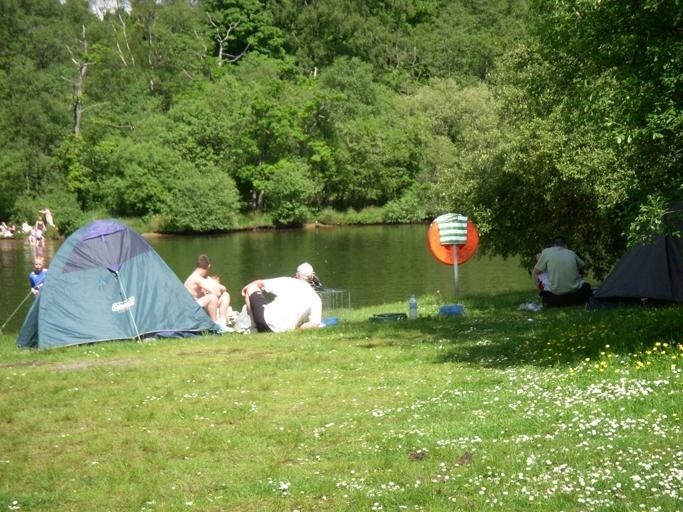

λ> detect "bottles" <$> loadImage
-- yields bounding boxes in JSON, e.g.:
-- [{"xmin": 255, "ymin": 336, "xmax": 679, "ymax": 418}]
[
  {"xmin": 409, "ymin": 293, "xmax": 418, "ymax": 320},
  {"xmin": 226, "ymin": 305, "xmax": 233, "ymax": 327}
]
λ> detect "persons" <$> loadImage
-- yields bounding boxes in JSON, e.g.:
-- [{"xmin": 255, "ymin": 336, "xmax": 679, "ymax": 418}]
[
  {"xmin": 1, "ymin": 206, "xmax": 58, "ymax": 297},
  {"xmin": 532, "ymin": 235, "xmax": 591, "ymax": 308},
  {"xmin": 183, "ymin": 253, "xmax": 235, "ymax": 335},
  {"xmin": 241, "ymin": 262, "xmax": 323, "ymax": 336}
]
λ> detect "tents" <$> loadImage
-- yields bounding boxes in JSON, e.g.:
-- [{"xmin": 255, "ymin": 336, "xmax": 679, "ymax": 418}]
[
  {"xmin": 15, "ymin": 218, "xmax": 220, "ymax": 352},
  {"xmin": 595, "ymin": 233, "xmax": 683, "ymax": 305}
]
